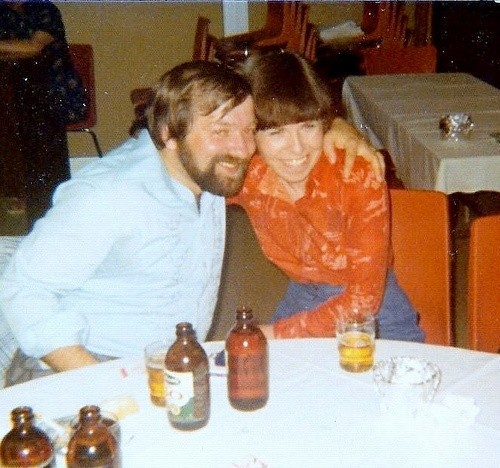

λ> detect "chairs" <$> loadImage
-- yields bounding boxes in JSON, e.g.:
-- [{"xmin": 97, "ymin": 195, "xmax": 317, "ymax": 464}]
[
  {"xmin": 203, "ymin": 203, "xmax": 291, "ymax": 341},
  {"xmin": 62, "ymin": 43, "xmax": 103, "ymax": 158},
  {"xmin": 468, "ymin": 215, "xmax": 500, "ymax": 354},
  {"xmin": 190, "ymin": 0, "xmax": 438, "ymax": 73},
  {"xmin": 388, "ymin": 189, "xmax": 453, "ymax": 346}
]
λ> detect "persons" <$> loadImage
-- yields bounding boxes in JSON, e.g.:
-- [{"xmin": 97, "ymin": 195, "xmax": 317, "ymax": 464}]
[
  {"xmin": 0, "ymin": 60, "xmax": 386, "ymax": 389},
  {"xmin": 0, "ymin": 0, "xmax": 72, "ymax": 235},
  {"xmin": 226, "ymin": 50, "xmax": 424, "ymax": 343}
]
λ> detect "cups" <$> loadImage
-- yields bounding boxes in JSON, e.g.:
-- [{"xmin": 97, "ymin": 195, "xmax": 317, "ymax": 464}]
[
  {"xmin": 336, "ymin": 308, "xmax": 375, "ymax": 373},
  {"xmin": 145, "ymin": 339, "xmax": 173, "ymax": 406},
  {"xmin": 373, "ymin": 356, "xmax": 440, "ymax": 405},
  {"xmin": 69, "ymin": 412, "xmax": 121, "ymax": 447}
]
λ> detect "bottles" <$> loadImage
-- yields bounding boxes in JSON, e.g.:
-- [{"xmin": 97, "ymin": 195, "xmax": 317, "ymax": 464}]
[
  {"xmin": 225, "ymin": 307, "xmax": 269, "ymax": 412},
  {"xmin": 164, "ymin": 322, "xmax": 211, "ymax": 432},
  {"xmin": 67, "ymin": 404, "xmax": 123, "ymax": 468},
  {"xmin": 0, "ymin": 406, "xmax": 57, "ymax": 468}
]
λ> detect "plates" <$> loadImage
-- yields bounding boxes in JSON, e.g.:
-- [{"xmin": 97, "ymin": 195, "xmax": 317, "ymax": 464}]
[{"xmin": 206, "ymin": 350, "xmax": 278, "ymax": 375}]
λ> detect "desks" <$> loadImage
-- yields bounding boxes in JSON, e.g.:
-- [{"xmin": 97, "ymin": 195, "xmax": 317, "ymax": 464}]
[
  {"xmin": 341, "ymin": 72, "xmax": 500, "ymax": 196},
  {"xmin": 0, "ymin": 337, "xmax": 500, "ymax": 467}
]
